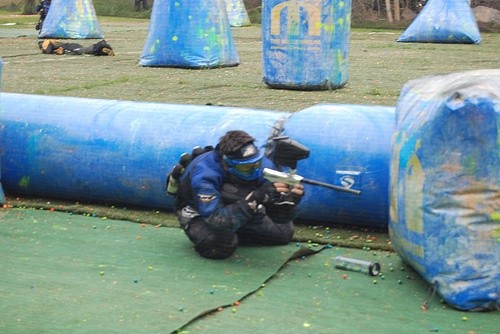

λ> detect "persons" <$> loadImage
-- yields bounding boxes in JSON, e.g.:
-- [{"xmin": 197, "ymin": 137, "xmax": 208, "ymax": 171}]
[
  {"xmin": 134, "ymin": 0, "xmax": 149, "ymax": 12},
  {"xmin": 34, "ymin": 0, "xmax": 52, "ymax": 31},
  {"xmin": 173, "ymin": 129, "xmax": 305, "ymax": 259},
  {"xmin": 37, "ymin": 39, "xmax": 115, "ymax": 57}
]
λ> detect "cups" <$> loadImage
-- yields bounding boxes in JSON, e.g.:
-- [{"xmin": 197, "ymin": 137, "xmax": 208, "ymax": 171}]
[{"xmin": 333, "ymin": 256, "xmax": 380, "ymax": 276}]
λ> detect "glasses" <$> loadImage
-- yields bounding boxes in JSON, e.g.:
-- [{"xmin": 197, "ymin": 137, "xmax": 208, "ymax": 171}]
[{"xmin": 221, "ymin": 155, "xmax": 264, "ymax": 177}]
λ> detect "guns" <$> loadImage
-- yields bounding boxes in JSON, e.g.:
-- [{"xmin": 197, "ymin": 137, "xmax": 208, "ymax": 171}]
[{"xmin": 222, "ymin": 128, "xmax": 363, "ymax": 215}]
